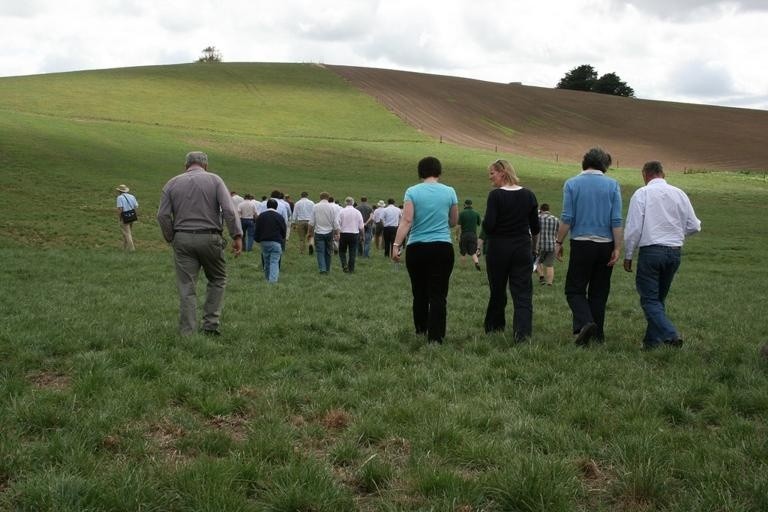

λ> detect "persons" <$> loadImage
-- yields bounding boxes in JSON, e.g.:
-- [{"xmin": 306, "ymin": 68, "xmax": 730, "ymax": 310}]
[
  {"xmin": 158, "ymin": 151, "xmax": 244, "ymax": 336},
  {"xmin": 231, "ymin": 190, "xmax": 565, "ymax": 286},
  {"xmin": 624, "ymin": 161, "xmax": 702, "ymax": 352},
  {"xmin": 116, "ymin": 184, "xmax": 139, "ymax": 251},
  {"xmin": 555, "ymin": 148, "xmax": 623, "ymax": 349},
  {"xmin": 392, "ymin": 158, "xmax": 459, "ymax": 344},
  {"xmin": 483, "ymin": 160, "xmax": 541, "ymax": 343}
]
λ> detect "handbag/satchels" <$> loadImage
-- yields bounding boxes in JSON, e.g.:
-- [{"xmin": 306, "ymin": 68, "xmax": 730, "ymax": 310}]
[{"xmin": 121, "ymin": 209, "xmax": 137, "ymax": 223}]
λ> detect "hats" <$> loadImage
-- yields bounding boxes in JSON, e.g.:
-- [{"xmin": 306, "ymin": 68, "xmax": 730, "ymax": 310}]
[
  {"xmin": 465, "ymin": 200, "xmax": 472, "ymax": 205},
  {"xmin": 116, "ymin": 184, "xmax": 130, "ymax": 192},
  {"xmin": 377, "ymin": 200, "xmax": 386, "ymax": 207}
]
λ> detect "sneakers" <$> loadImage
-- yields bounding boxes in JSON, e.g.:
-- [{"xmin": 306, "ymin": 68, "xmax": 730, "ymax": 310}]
[
  {"xmin": 575, "ymin": 322, "xmax": 596, "ymax": 346},
  {"xmin": 476, "ymin": 263, "xmax": 481, "ymax": 272},
  {"xmin": 670, "ymin": 337, "xmax": 683, "ymax": 348}
]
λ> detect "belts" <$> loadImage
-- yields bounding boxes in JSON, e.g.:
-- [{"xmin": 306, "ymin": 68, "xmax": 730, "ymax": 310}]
[{"xmin": 175, "ymin": 229, "xmax": 219, "ymax": 234}]
